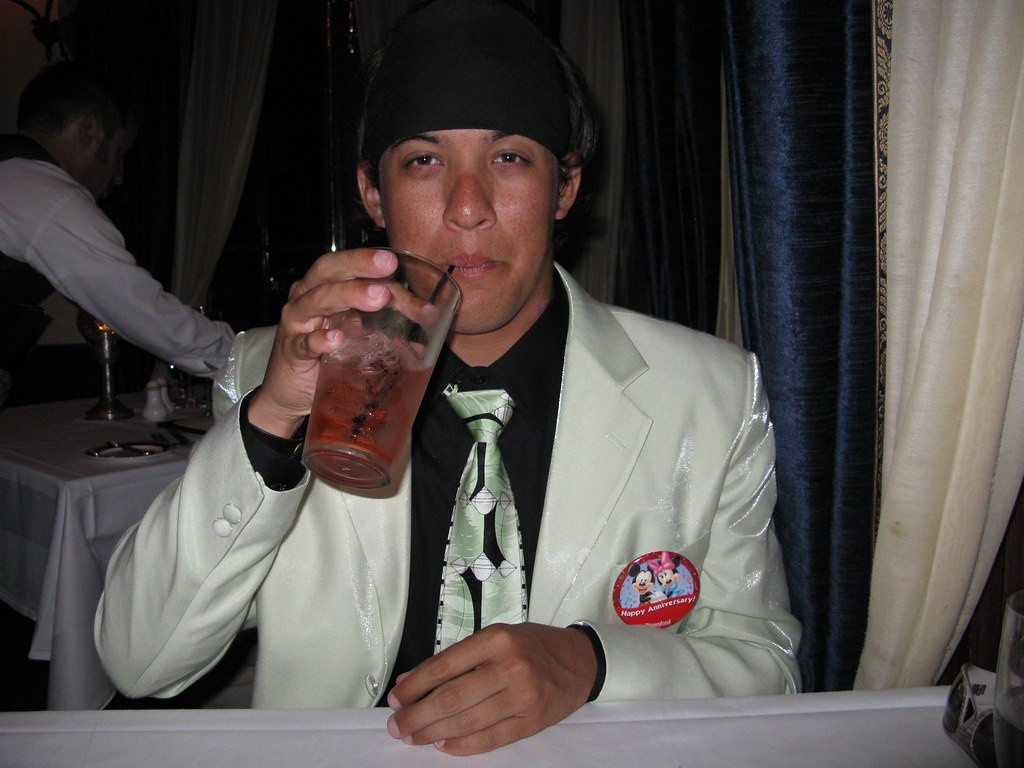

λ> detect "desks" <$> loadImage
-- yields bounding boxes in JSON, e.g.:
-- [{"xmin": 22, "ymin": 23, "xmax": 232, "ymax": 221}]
[{"xmin": 0, "ymin": 391, "xmax": 247, "ymax": 711}]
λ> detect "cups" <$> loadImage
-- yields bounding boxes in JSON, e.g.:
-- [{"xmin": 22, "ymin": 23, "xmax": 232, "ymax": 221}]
[
  {"xmin": 299, "ymin": 246, "xmax": 463, "ymax": 490},
  {"xmin": 992, "ymin": 589, "xmax": 1024, "ymax": 768}
]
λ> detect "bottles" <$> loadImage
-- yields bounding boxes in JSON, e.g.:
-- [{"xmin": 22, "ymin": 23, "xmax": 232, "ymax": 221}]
[{"xmin": 142, "ymin": 377, "xmax": 177, "ymax": 421}]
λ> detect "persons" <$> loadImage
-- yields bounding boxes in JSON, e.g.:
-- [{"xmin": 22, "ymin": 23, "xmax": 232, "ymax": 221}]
[
  {"xmin": 95, "ymin": 0, "xmax": 803, "ymax": 756},
  {"xmin": 1, "ymin": 61, "xmax": 235, "ymax": 379}
]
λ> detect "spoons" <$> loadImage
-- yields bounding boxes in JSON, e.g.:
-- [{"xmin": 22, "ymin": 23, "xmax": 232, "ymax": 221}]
[{"xmin": 105, "ymin": 439, "xmax": 154, "ymax": 455}]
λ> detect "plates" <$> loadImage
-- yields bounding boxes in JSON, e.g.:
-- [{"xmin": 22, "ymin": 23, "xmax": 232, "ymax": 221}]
[{"xmin": 83, "ymin": 442, "xmax": 164, "ymax": 461}]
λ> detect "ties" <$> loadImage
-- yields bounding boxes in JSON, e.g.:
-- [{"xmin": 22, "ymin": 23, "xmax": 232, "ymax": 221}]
[{"xmin": 433, "ymin": 383, "xmax": 528, "ymax": 655}]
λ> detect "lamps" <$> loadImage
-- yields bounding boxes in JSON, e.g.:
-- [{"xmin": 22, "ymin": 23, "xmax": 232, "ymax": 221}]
[{"xmin": 82, "ymin": 316, "xmax": 133, "ymax": 420}]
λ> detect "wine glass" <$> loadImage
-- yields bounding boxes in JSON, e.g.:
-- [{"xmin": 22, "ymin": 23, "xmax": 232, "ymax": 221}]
[{"xmin": 181, "ymin": 305, "xmax": 223, "ymax": 418}]
